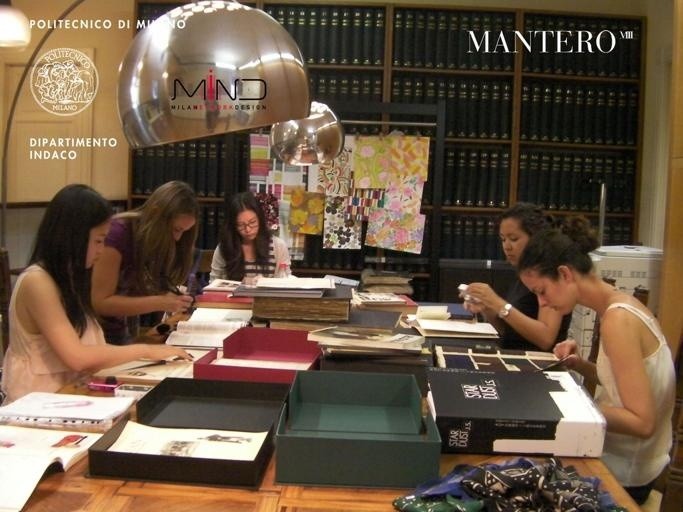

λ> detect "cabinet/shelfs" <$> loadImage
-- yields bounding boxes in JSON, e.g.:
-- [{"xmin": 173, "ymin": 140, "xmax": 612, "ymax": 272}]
[{"xmin": 125, "ymin": 4, "xmax": 647, "ymax": 316}]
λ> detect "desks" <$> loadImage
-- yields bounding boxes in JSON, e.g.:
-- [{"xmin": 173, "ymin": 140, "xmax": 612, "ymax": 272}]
[{"xmin": 0, "ymin": 296, "xmax": 641, "ymax": 512}]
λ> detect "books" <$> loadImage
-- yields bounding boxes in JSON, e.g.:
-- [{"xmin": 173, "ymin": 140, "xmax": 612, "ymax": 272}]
[{"xmin": 1, "ymin": 1, "xmax": 644, "ymax": 512}]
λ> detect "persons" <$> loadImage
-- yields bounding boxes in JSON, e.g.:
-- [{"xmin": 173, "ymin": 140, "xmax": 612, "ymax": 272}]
[{"xmin": 517, "ymin": 214, "xmax": 675, "ymax": 503}]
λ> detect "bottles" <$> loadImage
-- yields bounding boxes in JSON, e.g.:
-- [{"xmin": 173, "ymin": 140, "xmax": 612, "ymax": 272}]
[{"xmin": 275, "ymin": 262, "xmax": 289, "ymax": 277}]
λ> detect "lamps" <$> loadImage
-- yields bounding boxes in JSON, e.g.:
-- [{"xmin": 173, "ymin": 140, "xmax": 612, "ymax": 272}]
[
  {"xmin": 0, "ymin": 0, "xmax": 315, "ymax": 249},
  {"xmin": 269, "ymin": 100, "xmax": 344, "ymax": 170},
  {"xmin": 0, "ymin": 1, "xmax": 31, "ymax": 51}
]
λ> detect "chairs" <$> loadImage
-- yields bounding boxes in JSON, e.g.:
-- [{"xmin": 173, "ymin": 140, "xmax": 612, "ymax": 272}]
[
  {"xmin": 580, "ymin": 273, "xmax": 650, "ymax": 404},
  {"xmin": 0, "ymin": 245, "xmax": 27, "ymax": 361}
]
